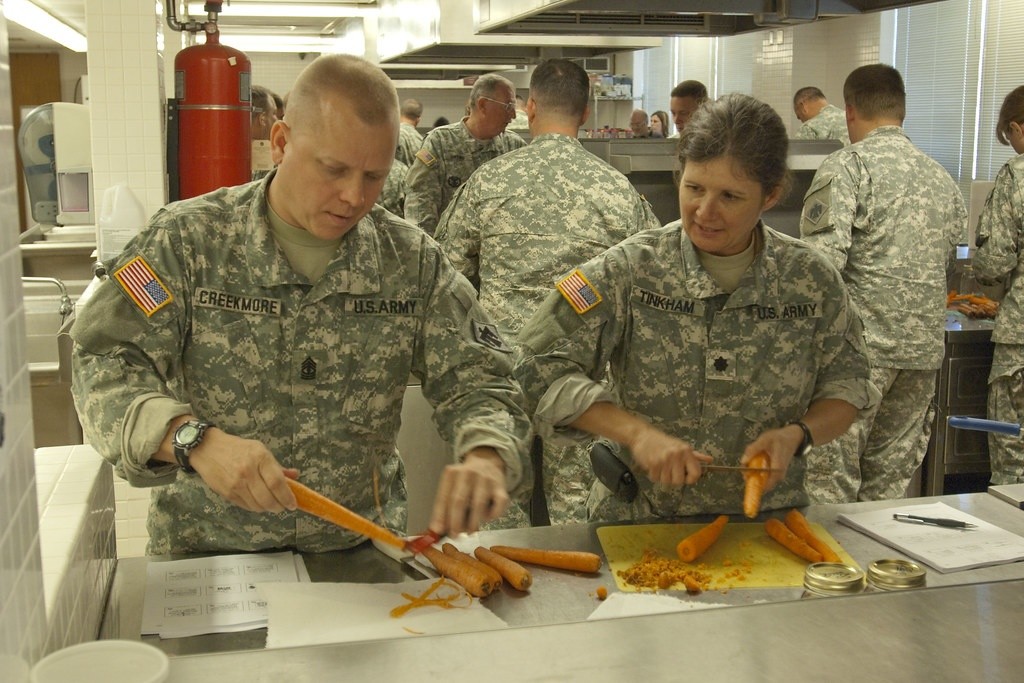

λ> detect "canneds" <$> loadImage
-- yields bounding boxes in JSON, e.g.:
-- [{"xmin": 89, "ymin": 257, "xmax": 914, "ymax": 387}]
[{"xmin": 586, "ymin": 125, "xmax": 630, "ymax": 138}]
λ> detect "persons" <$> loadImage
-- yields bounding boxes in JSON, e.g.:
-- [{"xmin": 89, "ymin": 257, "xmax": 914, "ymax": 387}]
[
  {"xmin": 510, "ymin": 94, "xmax": 881, "ymax": 522},
  {"xmin": 972, "ymin": 85, "xmax": 1024, "ymax": 486},
  {"xmin": 506, "ymin": 95, "xmax": 529, "ymax": 129},
  {"xmin": 794, "ymin": 86, "xmax": 851, "ymax": 148},
  {"xmin": 376, "ymin": 97, "xmax": 451, "ymax": 218},
  {"xmin": 69, "ymin": 54, "xmax": 533, "ymax": 554},
  {"xmin": 431, "ymin": 60, "xmax": 665, "ymax": 523},
  {"xmin": 630, "ymin": 109, "xmax": 669, "ymax": 139},
  {"xmin": 251, "ymin": 85, "xmax": 288, "ymax": 181},
  {"xmin": 800, "ymin": 66, "xmax": 970, "ymax": 506},
  {"xmin": 670, "ymin": 80, "xmax": 708, "ymax": 139},
  {"xmin": 403, "ymin": 73, "xmax": 529, "ymax": 237}
]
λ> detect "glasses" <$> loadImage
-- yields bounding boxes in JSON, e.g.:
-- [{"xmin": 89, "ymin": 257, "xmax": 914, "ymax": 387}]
[{"xmin": 482, "ymin": 96, "xmax": 514, "ymax": 114}]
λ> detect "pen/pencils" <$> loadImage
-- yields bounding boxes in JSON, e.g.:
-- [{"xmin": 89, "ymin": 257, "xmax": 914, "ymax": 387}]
[{"xmin": 893, "ymin": 513, "xmax": 978, "ymax": 528}]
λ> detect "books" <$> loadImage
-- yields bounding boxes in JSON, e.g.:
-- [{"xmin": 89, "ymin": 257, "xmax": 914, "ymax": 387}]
[{"xmin": 838, "ymin": 502, "xmax": 1024, "ymax": 573}]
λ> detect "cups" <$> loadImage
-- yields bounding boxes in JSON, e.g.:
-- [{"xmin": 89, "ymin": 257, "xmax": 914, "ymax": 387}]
[{"xmin": 29, "ymin": 639, "xmax": 171, "ymax": 682}]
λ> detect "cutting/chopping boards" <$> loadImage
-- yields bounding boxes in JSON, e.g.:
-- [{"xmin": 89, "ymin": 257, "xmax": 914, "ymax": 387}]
[{"xmin": 595, "ymin": 519, "xmax": 867, "ymax": 593}]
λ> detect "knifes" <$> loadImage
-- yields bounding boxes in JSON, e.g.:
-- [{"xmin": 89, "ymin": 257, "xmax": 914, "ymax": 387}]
[
  {"xmin": 700, "ymin": 463, "xmax": 782, "ymax": 474},
  {"xmin": 406, "ymin": 529, "xmax": 445, "ymax": 554}
]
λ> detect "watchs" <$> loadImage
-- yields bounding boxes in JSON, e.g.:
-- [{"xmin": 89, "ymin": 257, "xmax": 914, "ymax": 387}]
[
  {"xmin": 172, "ymin": 419, "xmax": 217, "ymax": 474},
  {"xmin": 781, "ymin": 421, "xmax": 814, "ymax": 458}
]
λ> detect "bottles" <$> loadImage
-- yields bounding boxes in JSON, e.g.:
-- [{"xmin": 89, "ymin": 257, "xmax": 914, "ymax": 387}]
[{"xmin": 600, "ymin": 72, "xmax": 632, "ymax": 98}]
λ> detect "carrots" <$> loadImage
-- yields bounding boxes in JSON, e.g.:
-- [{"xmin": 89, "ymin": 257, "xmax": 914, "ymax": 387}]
[
  {"xmin": 744, "ymin": 452, "xmax": 770, "ymax": 517},
  {"xmin": 677, "ymin": 515, "xmax": 728, "ymax": 562},
  {"xmin": 283, "ymin": 477, "xmax": 407, "ymax": 550},
  {"xmin": 943, "ymin": 292, "xmax": 999, "ymax": 308},
  {"xmin": 421, "ymin": 542, "xmax": 601, "ymax": 599},
  {"xmin": 785, "ymin": 510, "xmax": 843, "ymax": 563},
  {"xmin": 764, "ymin": 518, "xmax": 822, "ymax": 563}
]
